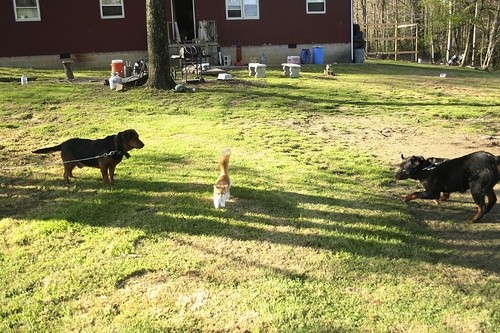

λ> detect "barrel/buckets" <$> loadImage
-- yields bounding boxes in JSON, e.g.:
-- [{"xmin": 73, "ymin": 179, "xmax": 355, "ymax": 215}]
[
  {"xmin": 312, "ymin": 47, "xmax": 324, "ymax": 65},
  {"xmin": 111, "ymin": 59, "xmax": 124, "ymax": 78},
  {"xmin": 124, "ymin": 66, "xmax": 132, "ymax": 78},
  {"xmin": 355, "ymin": 49, "xmax": 364, "ymax": 64},
  {"xmin": 249, "ymin": 56, "xmax": 259, "ymax": 63},
  {"xmin": 300, "ymin": 49, "xmax": 311, "ymax": 64}
]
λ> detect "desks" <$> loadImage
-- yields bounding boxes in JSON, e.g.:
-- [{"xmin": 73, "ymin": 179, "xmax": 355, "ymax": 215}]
[{"xmin": 171, "ymin": 55, "xmax": 211, "ymax": 81}]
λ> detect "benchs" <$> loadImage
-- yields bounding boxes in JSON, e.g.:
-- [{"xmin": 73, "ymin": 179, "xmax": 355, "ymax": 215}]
[
  {"xmin": 248, "ymin": 63, "xmax": 267, "ymax": 78},
  {"xmin": 281, "ymin": 63, "xmax": 301, "ymax": 77}
]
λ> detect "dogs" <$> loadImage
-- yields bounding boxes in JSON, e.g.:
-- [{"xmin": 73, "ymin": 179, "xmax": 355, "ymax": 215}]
[
  {"xmin": 134, "ymin": 59, "xmax": 146, "ymax": 75},
  {"xmin": 32, "ymin": 129, "xmax": 144, "ymax": 185},
  {"xmin": 323, "ymin": 64, "xmax": 335, "ymax": 77},
  {"xmin": 394, "ymin": 151, "xmax": 500, "ymax": 223}
]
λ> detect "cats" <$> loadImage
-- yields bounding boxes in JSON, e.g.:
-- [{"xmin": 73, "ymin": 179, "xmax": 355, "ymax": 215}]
[{"xmin": 213, "ymin": 153, "xmax": 232, "ymax": 209}]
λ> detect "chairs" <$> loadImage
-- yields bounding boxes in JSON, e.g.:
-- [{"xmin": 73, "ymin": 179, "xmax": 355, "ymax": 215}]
[{"xmin": 181, "ymin": 46, "xmax": 203, "ymax": 83}]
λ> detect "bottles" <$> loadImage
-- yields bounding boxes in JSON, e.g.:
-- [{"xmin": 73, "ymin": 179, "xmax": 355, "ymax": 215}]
[
  {"xmin": 21, "ymin": 75, "xmax": 27, "ymax": 85},
  {"xmin": 260, "ymin": 53, "xmax": 267, "ymax": 65}
]
